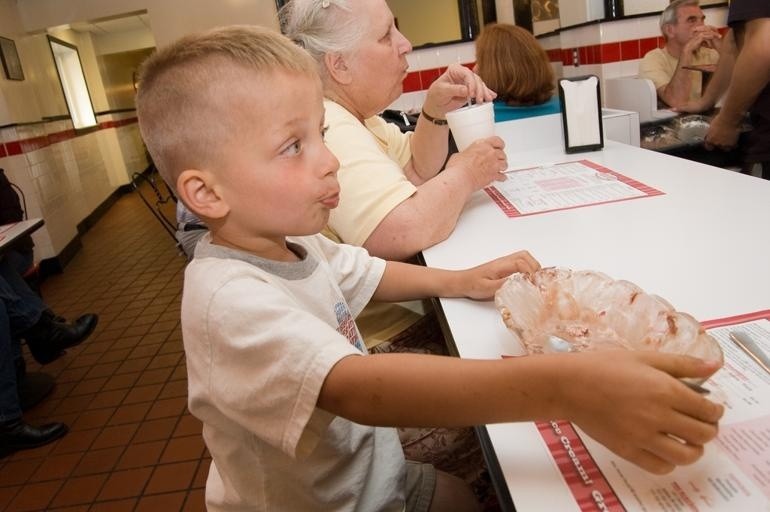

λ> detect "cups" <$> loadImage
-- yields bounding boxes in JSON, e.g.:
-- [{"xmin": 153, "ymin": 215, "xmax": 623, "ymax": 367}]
[{"xmin": 445, "ymin": 102, "xmax": 497, "ymax": 161}]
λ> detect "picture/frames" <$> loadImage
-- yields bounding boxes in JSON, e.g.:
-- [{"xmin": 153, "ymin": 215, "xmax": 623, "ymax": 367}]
[{"xmin": 0, "ymin": 36, "xmax": 25, "ymax": 81}]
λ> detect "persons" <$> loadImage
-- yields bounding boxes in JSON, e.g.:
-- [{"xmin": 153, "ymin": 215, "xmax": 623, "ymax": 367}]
[
  {"xmin": 637, "ymin": 0, "xmax": 725, "ymax": 109},
  {"xmin": 172, "ymin": 197, "xmax": 205, "ymax": 253},
  {"xmin": 275, "ymin": 1, "xmax": 512, "ymax": 480},
  {"xmin": 0, "ymin": 168, "xmax": 35, "ymax": 371},
  {"xmin": 1, "ymin": 253, "xmax": 99, "ymax": 456},
  {"xmin": 468, "ymin": 21, "xmax": 562, "ymax": 124},
  {"xmin": 673, "ymin": 1, "xmax": 770, "ymax": 179},
  {"xmin": 135, "ymin": 24, "xmax": 724, "ymax": 510}
]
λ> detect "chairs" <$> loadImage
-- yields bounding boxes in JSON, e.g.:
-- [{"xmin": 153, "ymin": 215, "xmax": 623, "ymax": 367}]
[
  {"xmin": 493, "ymin": 107, "xmax": 563, "ymax": 153},
  {"xmin": 132, "ymin": 162, "xmax": 193, "ymax": 264},
  {"xmin": 7, "ymin": 181, "xmax": 46, "ymax": 302},
  {"xmin": 603, "ymin": 74, "xmax": 679, "ymax": 124}
]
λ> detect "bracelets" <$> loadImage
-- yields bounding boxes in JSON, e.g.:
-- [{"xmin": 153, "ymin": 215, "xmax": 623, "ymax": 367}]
[{"xmin": 418, "ymin": 108, "xmax": 449, "ymax": 127}]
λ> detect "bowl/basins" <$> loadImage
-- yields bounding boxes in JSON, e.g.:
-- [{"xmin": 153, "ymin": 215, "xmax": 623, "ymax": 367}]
[{"xmin": 495, "ymin": 266, "xmax": 724, "ymax": 386}]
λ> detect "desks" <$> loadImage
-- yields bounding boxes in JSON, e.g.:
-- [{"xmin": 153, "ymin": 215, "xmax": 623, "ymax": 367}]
[
  {"xmin": 412, "ymin": 138, "xmax": 770, "ymax": 510},
  {"xmin": 683, "ymin": 63, "xmax": 719, "ymax": 113},
  {"xmin": 601, "ymin": 108, "xmax": 641, "ymax": 147},
  {"xmin": 1, "ymin": 219, "xmax": 57, "ymax": 411}
]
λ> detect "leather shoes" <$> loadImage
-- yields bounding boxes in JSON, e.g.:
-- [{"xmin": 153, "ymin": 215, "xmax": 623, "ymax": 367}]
[
  {"xmin": 28, "ymin": 313, "xmax": 99, "ymax": 365},
  {"xmin": 2, "ymin": 421, "xmax": 68, "ymax": 458}
]
